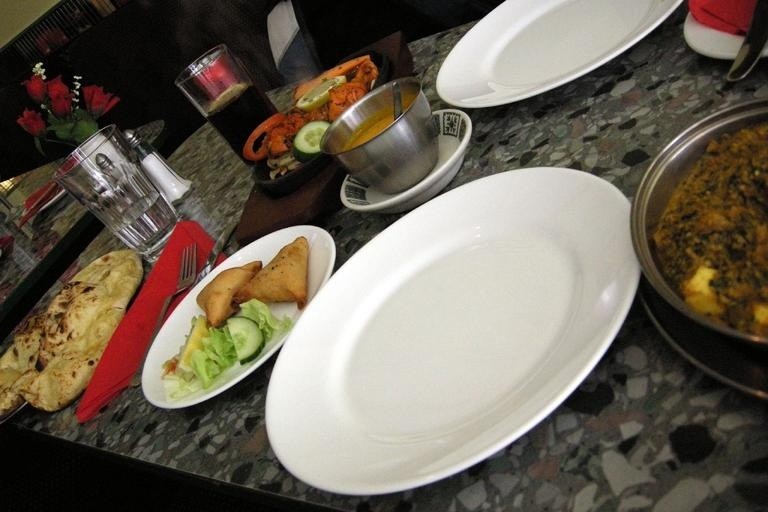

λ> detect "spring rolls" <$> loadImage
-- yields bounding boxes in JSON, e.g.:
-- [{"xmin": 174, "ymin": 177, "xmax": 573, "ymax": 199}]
[{"xmin": 197, "ymin": 235, "xmax": 310, "ymax": 327}]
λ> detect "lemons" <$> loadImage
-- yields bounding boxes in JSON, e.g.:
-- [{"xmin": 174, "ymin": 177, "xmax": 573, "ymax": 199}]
[
  {"xmin": 295, "ymin": 76, "xmax": 346, "ymax": 111},
  {"xmin": 173, "ymin": 313, "xmax": 206, "ymax": 375}
]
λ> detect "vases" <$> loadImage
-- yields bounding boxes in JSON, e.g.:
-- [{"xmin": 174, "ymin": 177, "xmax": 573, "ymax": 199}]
[{"xmin": 80, "ymin": 129, "xmax": 138, "ymax": 198}]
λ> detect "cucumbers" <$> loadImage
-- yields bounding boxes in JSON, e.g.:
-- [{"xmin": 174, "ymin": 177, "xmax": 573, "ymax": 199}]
[
  {"xmin": 224, "ymin": 318, "xmax": 265, "ymax": 364},
  {"xmin": 293, "ymin": 120, "xmax": 329, "ymax": 163}
]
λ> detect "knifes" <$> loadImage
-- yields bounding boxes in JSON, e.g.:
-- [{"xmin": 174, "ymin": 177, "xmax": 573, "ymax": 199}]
[
  {"xmin": 191, "ymin": 224, "xmax": 239, "ymax": 292},
  {"xmin": 727, "ymin": 1, "xmax": 768, "ymax": 83}
]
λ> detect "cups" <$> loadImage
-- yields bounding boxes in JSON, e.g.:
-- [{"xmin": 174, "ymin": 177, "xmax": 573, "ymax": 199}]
[
  {"xmin": 173, "ymin": 42, "xmax": 282, "ymax": 168},
  {"xmin": 48, "ymin": 124, "xmax": 183, "ymax": 263}
]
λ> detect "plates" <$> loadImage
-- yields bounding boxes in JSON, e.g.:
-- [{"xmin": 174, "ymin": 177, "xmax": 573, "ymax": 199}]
[
  {"xmin": 435, "ymin": 1, "xmax": 687, "ymax": 109},
  {"xmin": 141, "ymin": 223, "xmax": 339, "ymax": 412},
  {"xmin": 265, "ymin": 167, "xmax": 643, "ymax": 498},
  {"xmin": 340, "ymin": 108, "xmax": 473, "ymax": 216},
  {"xmin": 682, "ymin": 0, "xmax": 768, "ymax": 61}
]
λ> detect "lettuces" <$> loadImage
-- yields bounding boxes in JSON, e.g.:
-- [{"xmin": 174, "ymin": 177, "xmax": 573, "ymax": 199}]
[{"xmin": 187, "ymin": 299, "xmax": 290, "ymax": 388}]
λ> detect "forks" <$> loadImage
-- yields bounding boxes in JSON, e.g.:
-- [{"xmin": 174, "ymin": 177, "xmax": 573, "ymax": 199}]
[{"xmin": 129, "ymin": 241, "xmax": 197, "ymax": 386}]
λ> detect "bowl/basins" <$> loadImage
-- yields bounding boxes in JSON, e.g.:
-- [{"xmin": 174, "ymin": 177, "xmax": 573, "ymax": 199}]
[
  {"xmin": 319, "ymin": 76, "xmax": 439, "ymax": 194},
  {"xmin": 630, "ymin": 96, "xmax": 767, "ymax": 399}
]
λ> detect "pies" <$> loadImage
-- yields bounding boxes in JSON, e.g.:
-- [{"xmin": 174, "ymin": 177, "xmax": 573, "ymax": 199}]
[{"xmin": 1, "ymin": 244, "xmax": 145, "ymax": 416}]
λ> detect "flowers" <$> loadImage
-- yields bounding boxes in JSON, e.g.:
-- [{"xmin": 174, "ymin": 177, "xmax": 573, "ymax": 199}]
[{"xmin": 18, "ymin": 59, "xmax": 120, "ymax": 145}]
